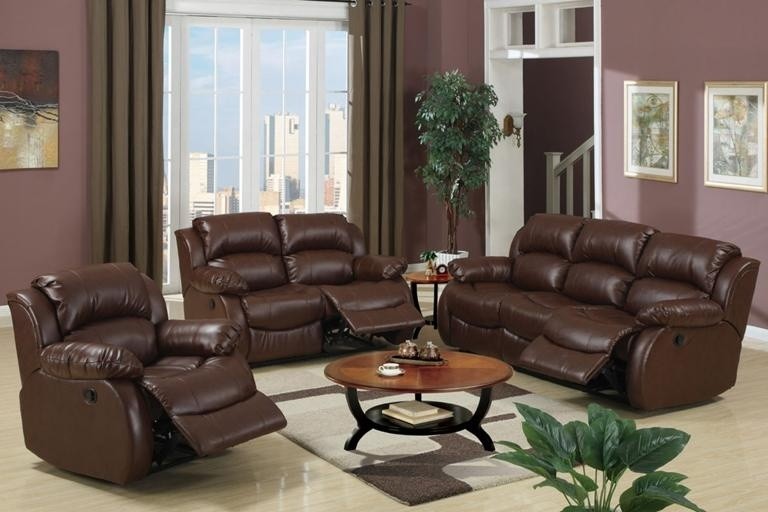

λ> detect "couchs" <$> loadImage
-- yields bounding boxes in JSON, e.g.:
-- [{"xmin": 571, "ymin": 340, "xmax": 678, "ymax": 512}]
[
  {"xmin": 442, "ymin": 208, "xmax": 761, "ymax": 411},
  {"xmin": 172, "ymin": 209, "xmax": 428, "ymax": 367}
]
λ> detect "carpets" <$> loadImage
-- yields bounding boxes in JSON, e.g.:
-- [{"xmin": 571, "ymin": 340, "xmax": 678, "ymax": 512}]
[{"xmin": 255, "ymin": 370, "xmax": 618, "ymax": 507}]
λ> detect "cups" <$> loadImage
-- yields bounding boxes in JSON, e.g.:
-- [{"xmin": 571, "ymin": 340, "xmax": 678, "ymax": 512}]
[{"xmin": 378, "ymin": 363, "xmax": 400, "ymax": 374}]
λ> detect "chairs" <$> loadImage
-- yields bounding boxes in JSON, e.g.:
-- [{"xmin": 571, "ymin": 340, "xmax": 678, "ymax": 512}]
[{"xmin": 6, "ymin": 256, "xmax": 287, "ymax": 489}]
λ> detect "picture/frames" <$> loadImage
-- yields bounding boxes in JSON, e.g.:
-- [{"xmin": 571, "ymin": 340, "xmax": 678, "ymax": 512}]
[
  {"xmin": 704, "ymin": 80, "xmax": 767, "ymax": 193},
  {"xmin": 623, "ymin": 80, "xmax": 678, "ymax": 182}
]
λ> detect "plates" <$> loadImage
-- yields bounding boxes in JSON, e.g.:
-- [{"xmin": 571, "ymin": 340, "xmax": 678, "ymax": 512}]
[{"xmin": 376, "ymin": 367, "xmax": 406, "ymax": 377}]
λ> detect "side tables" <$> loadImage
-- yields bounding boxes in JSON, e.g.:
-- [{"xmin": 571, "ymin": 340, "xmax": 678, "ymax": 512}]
[{"xmin": 406, "ymin": 271, "xmax": 450, "ymax": 324}]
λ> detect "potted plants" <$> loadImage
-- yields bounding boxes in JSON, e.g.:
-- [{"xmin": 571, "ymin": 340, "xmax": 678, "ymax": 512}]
[{"xmin": 410, "ymin": 62, "xmax": 506, "ymax": 273}]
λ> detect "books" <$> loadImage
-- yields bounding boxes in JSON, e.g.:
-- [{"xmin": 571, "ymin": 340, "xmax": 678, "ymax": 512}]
[{"xmin": 382, "ymin": 401, "xmax": 454, "ymax": 425}]
